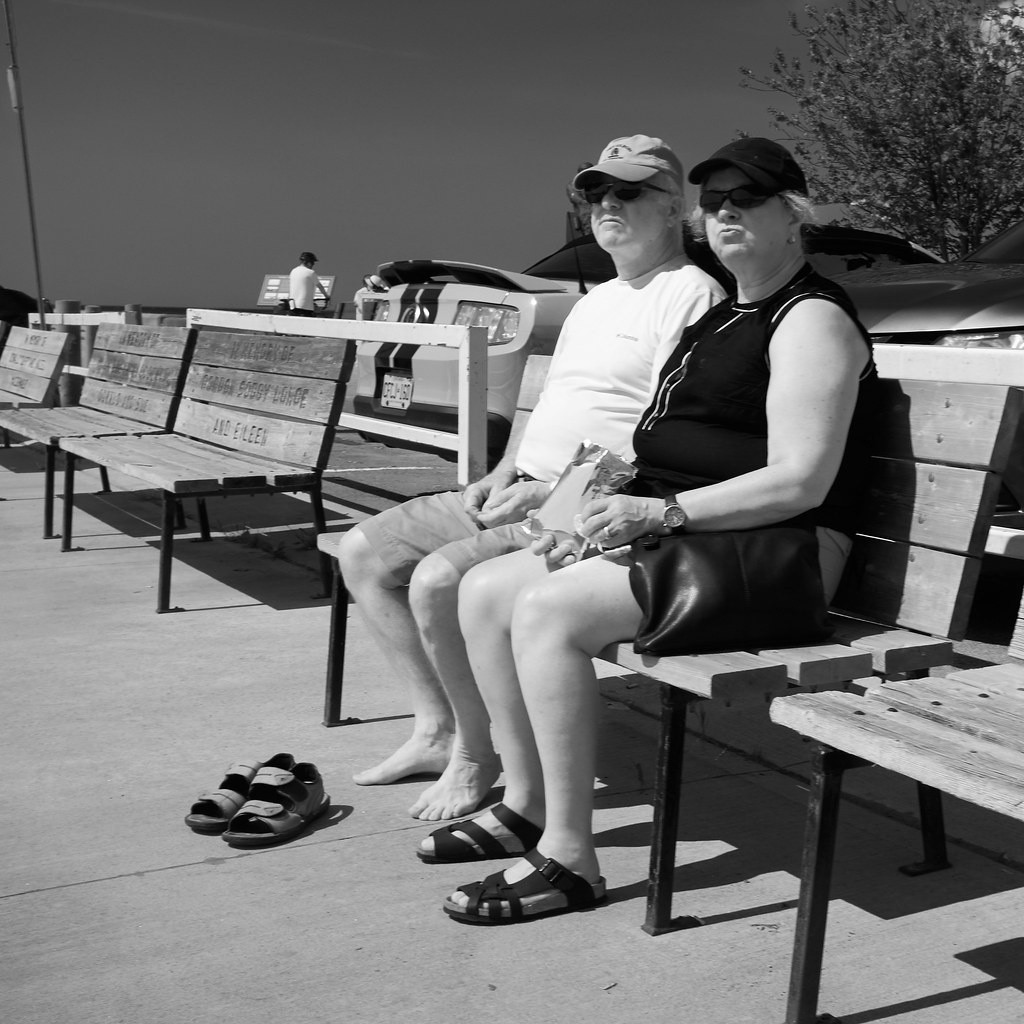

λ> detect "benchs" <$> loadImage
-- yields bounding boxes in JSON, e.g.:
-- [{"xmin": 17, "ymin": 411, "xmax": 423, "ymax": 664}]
[
  {"xmin": 767, "ymin": 595, "xmax": 1024, "ymax": 1024},
  {"xmin": 316, "ymin": 353, "xmax": 1023, "ymax": 935},
  {"xmin": 0, "ymin": 325, "xmax": 75, "ymax": 454},
  {"xmin": 58, "ymin": 330, "xmax": 357, "ymax": 615},
  {"xmin": 0, "ymin": 321, "xmax": 197, "ymax": 540}
]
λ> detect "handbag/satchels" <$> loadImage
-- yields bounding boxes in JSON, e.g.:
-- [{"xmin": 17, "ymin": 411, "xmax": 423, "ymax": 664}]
[{"xmin": 627, "ymin": 523, "xmax": 837, "ymax": 658}]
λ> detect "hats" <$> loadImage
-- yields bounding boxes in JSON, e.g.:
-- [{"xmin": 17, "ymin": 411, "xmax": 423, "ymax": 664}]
[
  {"xmin": 573, "ymin": 134, "xmax": 684, "ymax": 196},
  {"xmin": 688, "ymin": 137, "xmax": 809, "ymax": 199},
  {"xmin": 365, "ymin": 275, "xmax": 382, "ymax": 287}
]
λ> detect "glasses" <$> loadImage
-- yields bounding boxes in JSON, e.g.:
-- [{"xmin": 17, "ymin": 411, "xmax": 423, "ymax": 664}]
[
  {"xmin": 583, "ymin": 178, "xmax": 672, "ymax": 204},
  {"xmin": 700, "ymin": 183, "xmax": 774, "ymax": 213}
]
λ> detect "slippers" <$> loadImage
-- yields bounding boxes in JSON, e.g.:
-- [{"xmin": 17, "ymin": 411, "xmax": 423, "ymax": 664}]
[
  {"xmin": 443, "ymin": 848, "xmax": 606, "ymax": 922},
  {"xmin": 416, "ymin": 802, "xmax": 543, "ymax": 864}
]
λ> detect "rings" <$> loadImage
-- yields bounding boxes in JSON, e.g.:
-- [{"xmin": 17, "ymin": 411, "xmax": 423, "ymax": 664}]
[{"xmin": 604, "ymin": 527, "xmax": 613, "ymax": 539}]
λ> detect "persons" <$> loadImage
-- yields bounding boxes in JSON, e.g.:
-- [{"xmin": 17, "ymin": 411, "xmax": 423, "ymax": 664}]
[
  {"xmin": 337, "ymin": 132, "xmax": 728, "ymax": 823},
  {"xmin": 288, "ymin": 252, "xmax": 331, "ymax": 317},
  {"xmin": 417, "ymin": 137, "xmax": 882, "ymax": 923}
]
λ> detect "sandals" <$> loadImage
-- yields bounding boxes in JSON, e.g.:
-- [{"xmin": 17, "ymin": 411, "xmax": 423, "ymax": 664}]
[{"xmin": 184, "ymin": 753, "xmax": 330, "ymax": 844}]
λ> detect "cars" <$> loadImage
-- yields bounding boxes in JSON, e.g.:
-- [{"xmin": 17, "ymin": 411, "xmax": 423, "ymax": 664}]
[
  {"xmin": 824, "ymin": 217, "xmax": 1024, "ymax": 532},
  {"xmin": 345, "ymin": 222, "xmax": 947, "ymax": 475}
]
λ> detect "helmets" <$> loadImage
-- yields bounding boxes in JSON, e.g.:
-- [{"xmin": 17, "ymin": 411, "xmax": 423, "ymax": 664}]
[{"xmin": 299, "ymin": 252, "xmax": 317, "ymax": 262}]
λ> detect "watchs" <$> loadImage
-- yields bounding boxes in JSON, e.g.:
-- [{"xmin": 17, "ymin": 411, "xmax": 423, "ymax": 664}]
[{"xmin": 663, "ymin": 494, "xmax": 688, "ymax": 535}]
